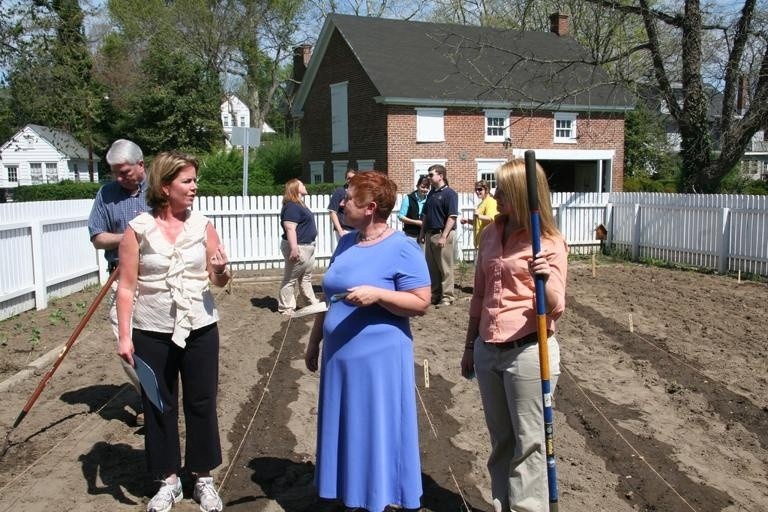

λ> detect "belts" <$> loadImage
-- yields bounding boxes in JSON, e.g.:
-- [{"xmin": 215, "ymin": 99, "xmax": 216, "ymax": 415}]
[
  {"xmin": 426, "ymin": 228, "xmax": 453, "ymax": 234},
  {"xmin": 485, "ymin": 329, "xmax": 551, "ymax": 350}
]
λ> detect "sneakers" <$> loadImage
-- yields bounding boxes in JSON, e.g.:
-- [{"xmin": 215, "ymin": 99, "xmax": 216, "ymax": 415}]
[
  {"xmin": 430, "ymin": 295, "xmax": 441, "ymax": 305},
  {"xmin": 436, "ymin": 296, "xmax": 455, "ymax": 308},
  {"xmin": 278, "ymin": 306, "xmax": 299, "ymax": 317},
  {"xmin": 136, "ymin": 400, "xmax": 145, "ymax": 427},
  {"xmin": 147, "ymin": 473, "xmax": 185, "ymax": 511},
  {"xmin": 192, "ymin": 473, "xmax": 223, "ymax": 512}
]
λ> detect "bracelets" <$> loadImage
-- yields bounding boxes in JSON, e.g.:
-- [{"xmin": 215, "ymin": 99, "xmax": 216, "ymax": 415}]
[
  {"xmin": 215, "ymin": 269, "xmax": 227, "ymax": 277},
  {"xmin": 465, "ymin": 339, "xmax": 475, "ymax": 345},
  {"xmin": 465, "ymin": 346, "xmax": 473, "ymax": 350}
]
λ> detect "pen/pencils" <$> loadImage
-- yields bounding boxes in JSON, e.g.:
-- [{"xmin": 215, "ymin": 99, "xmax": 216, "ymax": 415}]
[{"xmin": 330, "ymin": 292, "xmax": 349, "ymax": 303}]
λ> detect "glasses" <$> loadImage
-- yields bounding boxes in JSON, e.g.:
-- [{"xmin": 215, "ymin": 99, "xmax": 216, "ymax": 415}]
[
  {"xmin": 344, "ymin": 188, "xmax": 356, "ymax": 204},
  {"xmin": 427, "ymin": 173, "xmax": 440, "ymax": 178},
  {"xmin": 475, "ymin": 187, "xmax": 487, "ymax": 192}
]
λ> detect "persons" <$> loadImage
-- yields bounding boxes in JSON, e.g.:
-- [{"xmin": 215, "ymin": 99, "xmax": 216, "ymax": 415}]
[
  {"xmin": 326, "ymin": 170, "xmax": 360, "ymax": 244},
  {"xmin": 276, "ymin": 179, "xmax": 324, "ymax": 317},
  {"xmin": 85, "ymin": 138, "xmax": 154, "ymax": 428},
  {"xmin": 460, "ymin": 155, "xmax": 571, "ymax": 512},
  {"xmin": 304, "ymin": 172, "xmax": 435, "ymax": 512},
  {"xmin": 114, "ymin": 149, "xmax": 231, "ymax": 512},
  {"xmin": 417, "ymin": 164, "xmax": 460, "ymax": 309},
  {"xmin": 395, "ymin": 174, "xmax": 436, "ymax": 255},
  {"xmin": 458, "ymin": 179, "xmax": 499, "ymax": 264}
]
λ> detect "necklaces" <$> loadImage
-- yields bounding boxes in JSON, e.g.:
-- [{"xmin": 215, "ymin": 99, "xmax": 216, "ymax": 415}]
[{"xmin": 358, "ymin": 223, "xmax": 390, "ymax": 242}]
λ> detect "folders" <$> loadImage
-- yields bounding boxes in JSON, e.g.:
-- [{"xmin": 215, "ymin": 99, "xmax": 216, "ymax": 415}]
[{"xmin": 132, "ymin": 354, "xmax": 165, "ymax": 414}]
